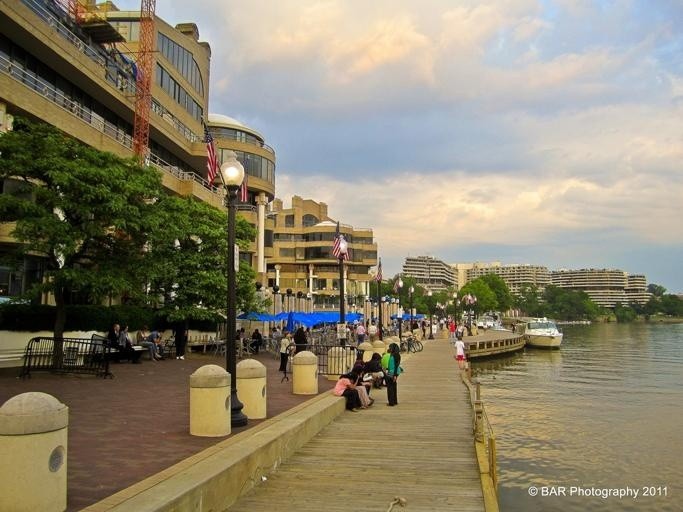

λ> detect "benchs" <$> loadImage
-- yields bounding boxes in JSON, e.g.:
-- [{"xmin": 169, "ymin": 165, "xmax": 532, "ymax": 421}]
[
  {"xmin": 105, "ymin": 346, "xmax": 148, "ymax": 357},
  {"xmin": 0, "ymin": 348, "xmax": 89, "ymax": 361}
]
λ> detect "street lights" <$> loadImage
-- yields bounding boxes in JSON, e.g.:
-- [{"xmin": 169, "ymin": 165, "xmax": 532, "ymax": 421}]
[
  {"xmin": 218, "ymin": 150, "xmax": 248, "ymax": 428},
  {"xmin": 453, "ymin": 292, "xmax": 457, "ymax": 328},
  {"xmin": 376, "ymin": 268, "xmax": 383, "ymax": 340},
  {"xmin": 398, "ymin": 277, "xmax": 406, "ymax": 352},
  {"xmin": 427, "ymin": 289, "xmax": 434, "ymax": 339},
  {"xmin": 339, "ymin": 234, "xmax": 346, "ymax": 350},
  {"xmin": 409, "ymin": 285, "xmax": 414, "ymax": 333}
]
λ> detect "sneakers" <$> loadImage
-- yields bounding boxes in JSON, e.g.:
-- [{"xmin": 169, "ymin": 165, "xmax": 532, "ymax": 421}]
[
  {"xmin": 149, "ymin": 353, "xmax": 165, "ymax": 361},
  {"xmin": 177, "ymin": 356, "xmax": 184, "ymax": 360}
]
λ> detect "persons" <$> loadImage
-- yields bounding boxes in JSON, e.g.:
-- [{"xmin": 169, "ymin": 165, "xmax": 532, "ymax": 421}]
[
  {"xmin": 232, "ymin": 313, "xmax": 468, "ymax": 410},
  {"xmin": 108, "ymin": 320, "xmax": 191, "ymax": 363}
]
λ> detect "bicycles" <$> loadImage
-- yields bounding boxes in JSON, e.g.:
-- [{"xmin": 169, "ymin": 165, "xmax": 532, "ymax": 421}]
[{"xmin": 400, "ymin": 334, "xmax": 423, "ymax": 353}]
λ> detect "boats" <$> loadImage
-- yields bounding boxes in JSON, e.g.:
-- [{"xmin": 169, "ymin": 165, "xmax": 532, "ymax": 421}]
[{"xmin": 524, "ymin": 317, "xmax": 563, "ymax": 350}]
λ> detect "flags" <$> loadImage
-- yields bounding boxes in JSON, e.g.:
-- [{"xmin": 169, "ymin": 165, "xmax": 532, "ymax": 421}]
[
  {"xmin": 333, "ymin": 227, "xmax": 343, "ymax": 259},
  {"xmin": 393, "ymin": 277, "xmax": 401, "ymax": 295},
  {"xmin": 241, "ymin": 157, "xmax": 251, "ymax": 204},
  {"xmin": 376, "ymin": 258, "xmax": 384, "ymax": 284},
  {"xmin": 202, "ymin": 129, "xmax": 218, "ymax": 185},
  {"xmin": 343, "ymin": 230, "xmax": 351, "ymax": 262}
]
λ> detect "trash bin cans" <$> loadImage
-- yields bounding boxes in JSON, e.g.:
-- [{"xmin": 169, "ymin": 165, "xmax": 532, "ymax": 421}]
[
  {"xmin": 471, "ymin": 325, "xmax": 479, "ymax": 337},
  {"xmin": 327, "ymin": 340, "xmax": 384, "ymax": 381},
  {"xmin": 385, "ymin": 329, "xmax": 423, "ymax": 355},
  {"xmin": 463, "ymin": 327, "xmax": 467, "ymax": 336},
  {"xmin": 294, "ymin": 351, "xmax": 318, "ymax": 395},
  {"xmin": 235, "ymin": 359, "xmax": 267, "ymax": 419},
  {"xmin": 1, "ymin": 391, "xmax": 68, "ymax": 512},
  {"xmin": 441, "ymin": 328, "xmax": 448, "ymax": 340},
  {"xmin": 189, "ymin": 364, "xmax": 232, "ymax": 437}
]
